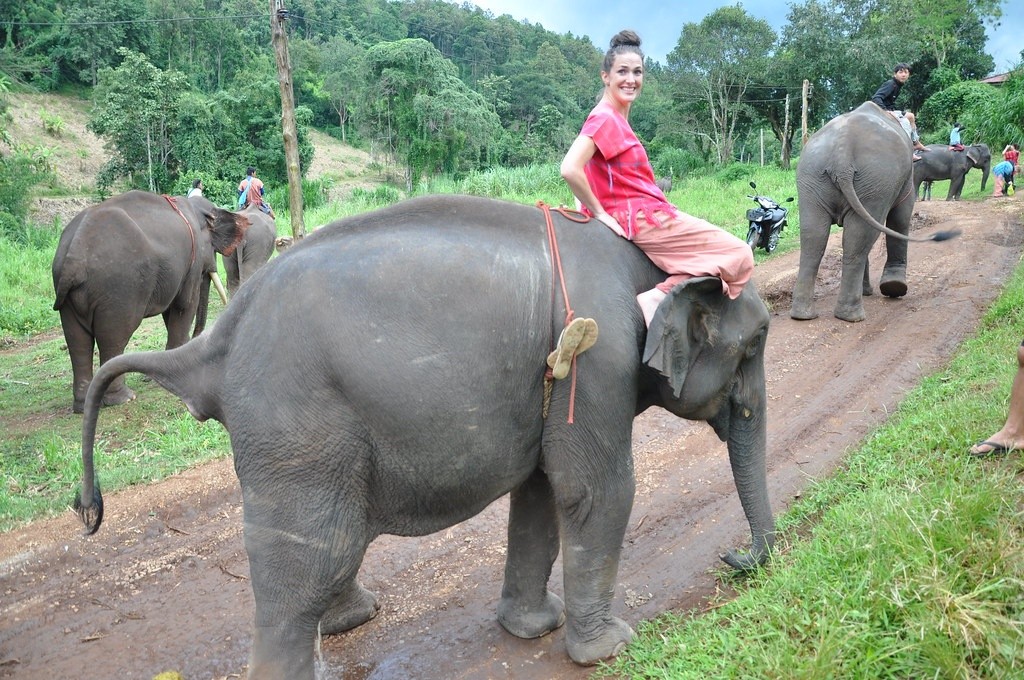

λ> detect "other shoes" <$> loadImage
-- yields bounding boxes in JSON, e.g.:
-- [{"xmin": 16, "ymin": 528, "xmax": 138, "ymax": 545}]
[{"xmin": 268, "ymin": 212, "xmax": 275, "ymax": 220}]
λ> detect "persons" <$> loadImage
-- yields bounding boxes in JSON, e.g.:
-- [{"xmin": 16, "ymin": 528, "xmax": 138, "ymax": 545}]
[
  {"xmin": 993, "ymin": 144, "xmax": 1020, "ymax": 197},
  {"xmin": 949, "ymin": 122, "xmax": 965, "ymax": 151},
  {"xmin": 873, "ymin": 62, "xmax": 931, "ymax": 162},
  {"xmin": 561, "ymin": 31, "xmax": 754, "ymax": 330},
  {"xmin": 238, "ymin": 167, "xmax": 275, "ymax": 219},
  {"xmin": 967, "ymin": 340, "xmax": 1024, "ymax": 456},
  {"xmin": 188, "ymin": 179, "xmax": 202, "ymax": 198}
]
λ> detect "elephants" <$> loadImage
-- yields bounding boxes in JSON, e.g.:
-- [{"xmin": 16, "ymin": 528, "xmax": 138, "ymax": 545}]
[
  {"xmin": 53, "ymin": 188, "xmax": 249, "ymax": 414},
  {"xmin": 913, "ymin": 143, "xmax": 992, "ymax": 202},
  {"xmin": 72, "ymin": 195, "xmax": 774, "ymax": 680},
  {"xmin": 788, "ymin": 101, "xmax": 963, "ymax": 322},
  {"xmin": 221, "ymin": 200, "xmax": 275, "ymax": 304}
]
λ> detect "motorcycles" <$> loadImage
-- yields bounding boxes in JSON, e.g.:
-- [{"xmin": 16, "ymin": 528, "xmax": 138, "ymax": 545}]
[{"xmin": 746, "ymin": 181, "xmax": 795, "ymax": 253}]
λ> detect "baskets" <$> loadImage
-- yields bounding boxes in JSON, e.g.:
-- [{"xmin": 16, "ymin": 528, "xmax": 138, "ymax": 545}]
[{"xmin": 746, "ymin": 209, "xmax": 764, "ymax": 222}]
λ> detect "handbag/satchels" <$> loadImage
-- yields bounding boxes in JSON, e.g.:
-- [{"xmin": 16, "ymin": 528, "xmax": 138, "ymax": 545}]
[
  {"xmin": 1007, "ymin": 184, "xmax": 1014, "ymax": 195},
  {"xmin": 238, "ymin": 181, "xmax": 251, "ymax": 205}
]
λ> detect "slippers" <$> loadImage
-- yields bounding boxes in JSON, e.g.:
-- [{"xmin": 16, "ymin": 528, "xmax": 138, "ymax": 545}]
[
  {"xmin": 969, "ymin": 441, "xmax": 1024, "ymax": 454},
  {"xmin": 547, "ymin": 318, "xmax": 599, "ymax": 380}
]
